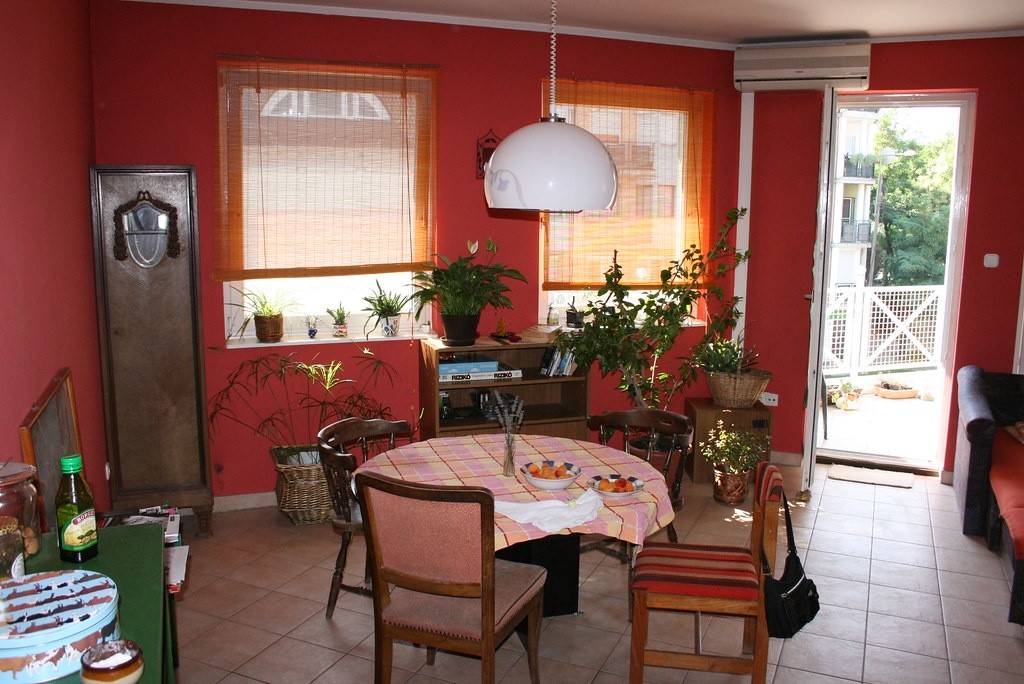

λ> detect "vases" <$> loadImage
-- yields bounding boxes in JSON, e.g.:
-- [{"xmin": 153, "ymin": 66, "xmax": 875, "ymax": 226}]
[
  {"xmin": 308, "ymin": 326, "xmax": 317, "ymax": 339},
  {"xmin": 685, "ymin": 398, "xmax": 775, "ymax": 483},
  {"xmin": 503, "ymin": 436, "xmax": 515, "ymax": 478}
]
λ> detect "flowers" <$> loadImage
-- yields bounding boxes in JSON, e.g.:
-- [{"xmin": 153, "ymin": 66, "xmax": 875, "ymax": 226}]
[
  {"xmin": 494, "ymin": 389, "xmax": 524, "ymax": 445},
  {"xmin": 303, "ymin": 314, "xmax": 322, "ymax": 331}
]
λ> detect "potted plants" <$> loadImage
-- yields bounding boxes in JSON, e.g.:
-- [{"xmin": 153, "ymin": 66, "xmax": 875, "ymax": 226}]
[
  {"xmin": 360, "ymin": 279, "xmax": 413, "ymax": 340},
  {"xmin": 327, "ymin": 302, "xmax": 353, "ymax": 335},
  {"xmin": 872, "ymin": 370, "xmax": 922, "ymax": 398},
  {"xmin": 209, "ymin": 346, "xmax": 393, "ymax": 523},
  {"xmin": 694, "ymin": 418, "xmax": 772, "ymax": 503},
  {"xmin": 223, "ymin": 282, "xmax": 307, "ymax": 345},
  {"xmin": 683, "ymin": 342, "xmax": 775, "ymax": 407},
  {"xmin": 825, "ymin": 380, "xmax": 865, "ymax": 410},
  {"xmin": 409, "ymin": 235, "xmax": 524, "ymax": 347}
]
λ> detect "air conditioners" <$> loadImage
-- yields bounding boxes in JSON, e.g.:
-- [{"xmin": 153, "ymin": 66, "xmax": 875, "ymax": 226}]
[{"xmin": 732, "ymin": 36, "xmax": 872, "ymax": 92}]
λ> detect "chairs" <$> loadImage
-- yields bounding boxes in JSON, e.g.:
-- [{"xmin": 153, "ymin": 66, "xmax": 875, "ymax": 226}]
[
  {"xmin": 356, "ymin": 470, "xmax": 548, "ymax": 683},
  {"xmin": 578, "ymin": 410, "xmax": 696, "ymax": 621},
  {"xmin": 318, "ymin": 417, "xmax": 411, "ymax": 619},
  {"xmin": 631, "ymin": 461, "xmax": 785, "ymax": 682}
]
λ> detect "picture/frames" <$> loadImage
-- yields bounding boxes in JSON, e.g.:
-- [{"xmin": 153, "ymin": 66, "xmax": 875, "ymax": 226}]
[{"xmin": 17, "ymin": 366, "xmax": 87, "ymax": 533}]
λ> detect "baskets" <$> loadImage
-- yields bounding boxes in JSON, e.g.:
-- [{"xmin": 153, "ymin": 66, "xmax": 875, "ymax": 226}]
[
  {"xmin": 269, "ymin": 443, "xmax": 337, "ymax": 526},
  {"xmin": 703, "ymin": 357, "xmax": 773, "ymax": 410}
]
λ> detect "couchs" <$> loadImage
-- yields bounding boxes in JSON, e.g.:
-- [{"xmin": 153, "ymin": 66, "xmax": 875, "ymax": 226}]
[{"xmin": 954, "ymin": 364, "xmax": 1024, "ymax": 626}]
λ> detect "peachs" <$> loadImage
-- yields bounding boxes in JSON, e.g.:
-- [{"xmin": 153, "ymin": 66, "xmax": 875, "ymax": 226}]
[
  {"xmin": 528, "ymin": 464, "xmax": 573, "ymax": 480},
  {"xmin": 599, "ymin": 478, "xmax": 635, "ymax": 492}
]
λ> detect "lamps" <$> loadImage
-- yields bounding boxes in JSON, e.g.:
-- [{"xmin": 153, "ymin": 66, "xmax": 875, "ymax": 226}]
[{"xmin": 479, "ymin": 1, "xmax": 617, "ymax": 213}]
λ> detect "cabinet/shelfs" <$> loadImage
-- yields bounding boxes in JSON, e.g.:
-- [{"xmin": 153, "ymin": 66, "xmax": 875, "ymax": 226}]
[
  {"xmin": 419, "ymin": 336, "xmax": 591, "ymax": 442},
  {"xmin": 24, "ymin": 523, "xmax": 177, "ymax": 683}
]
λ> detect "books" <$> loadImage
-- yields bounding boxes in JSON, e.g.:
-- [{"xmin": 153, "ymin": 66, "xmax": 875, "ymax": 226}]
[{"xmin": 539, "ymin": 346, "xmax": 581, "ymax": 378}]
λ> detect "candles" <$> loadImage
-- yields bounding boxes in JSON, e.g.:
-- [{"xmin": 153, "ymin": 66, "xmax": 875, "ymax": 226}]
[{"xmin": 555, "ymin": 201, "xmax": 752, "ymax": 503}]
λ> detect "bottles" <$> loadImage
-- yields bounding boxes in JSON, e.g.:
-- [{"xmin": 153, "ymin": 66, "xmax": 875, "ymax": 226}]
[
  {"xmin": 0, "ymin": 516, "xmax": 25, "ymax": 582},
  {"xmin": 547, "ymin": 306, "xmax": 559, "ymax": 326},
  {"xmin": 0, "ymin": 476, "xmax": 42, "ymax": 561},
  {"xmin": 55, "ymin": 454, "xmax": 99, "ymax": 562}
]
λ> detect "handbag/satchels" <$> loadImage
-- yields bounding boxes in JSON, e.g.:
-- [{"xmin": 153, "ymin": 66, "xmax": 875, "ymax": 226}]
[{"xmin": 763, "ymin": 549, "xmax": 820, "ymax": 639}]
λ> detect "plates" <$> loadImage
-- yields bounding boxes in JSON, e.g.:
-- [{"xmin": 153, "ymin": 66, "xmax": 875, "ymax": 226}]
[{"xmin": 587, "ymin": 473, "xmax": 644, "ymax": 497}]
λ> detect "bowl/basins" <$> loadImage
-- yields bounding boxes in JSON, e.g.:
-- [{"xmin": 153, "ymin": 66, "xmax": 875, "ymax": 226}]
[
  {"xmin": 79, "ymin": 639, "xmax": 144, "ymax": 684},
  {"xmin": 520, "ymin": 460, "xmax": 582, "ymax": 489}
]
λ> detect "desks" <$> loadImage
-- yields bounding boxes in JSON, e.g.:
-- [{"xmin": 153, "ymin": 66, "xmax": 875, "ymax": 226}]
[{"xmin": 349, "ymin": 433, "xmax": 678, "ymax": 621}]
